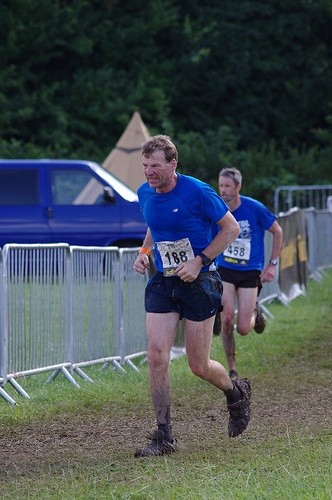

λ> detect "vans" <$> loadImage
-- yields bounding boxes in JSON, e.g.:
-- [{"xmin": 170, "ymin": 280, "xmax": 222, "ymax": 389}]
[{"xmin": 0, "ymin": 158, "xmax": 149, "ymax": 275}]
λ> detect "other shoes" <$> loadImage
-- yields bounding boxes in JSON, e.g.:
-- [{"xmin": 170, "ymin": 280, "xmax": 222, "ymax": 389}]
[
  {"xmin": 228, "ymin": 370, "xmax": 238, "ymax": 382},
  {"xmin": 254, "ymin": 309, "xmax": 265, "ymax": 334}
]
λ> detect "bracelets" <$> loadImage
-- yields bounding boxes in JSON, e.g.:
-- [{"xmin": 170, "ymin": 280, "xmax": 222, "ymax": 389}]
[{"xmin": 139, "ymin": 247, "xmax": 150, "ymax": 255}]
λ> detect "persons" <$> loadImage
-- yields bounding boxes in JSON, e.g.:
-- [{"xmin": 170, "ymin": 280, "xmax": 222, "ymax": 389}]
[
  {"xmin": 214, "ymin": 167, "xmax": 284, "ymax": 380},
  {"xmin": 133, "ymin": 134, "xmax": 252, "ymax": 458}
]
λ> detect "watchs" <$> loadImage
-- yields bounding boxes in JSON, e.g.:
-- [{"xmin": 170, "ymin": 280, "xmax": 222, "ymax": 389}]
[
  {"xmin": 269, "ymin": 257, "xmax": 278, "ymax": 265},
  {"xmin": 199, "ymin": 252, "xmax": 212, "ymax": 267}
]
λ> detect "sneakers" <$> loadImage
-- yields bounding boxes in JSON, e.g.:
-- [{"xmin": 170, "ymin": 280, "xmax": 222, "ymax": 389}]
[
  {"xmin": 226, "ymin": 378, "xmax": 252, "ymax": 438},
  {"xmin": 135, "ymin": 430, "xmax": 178, "ymax": 458}
]
